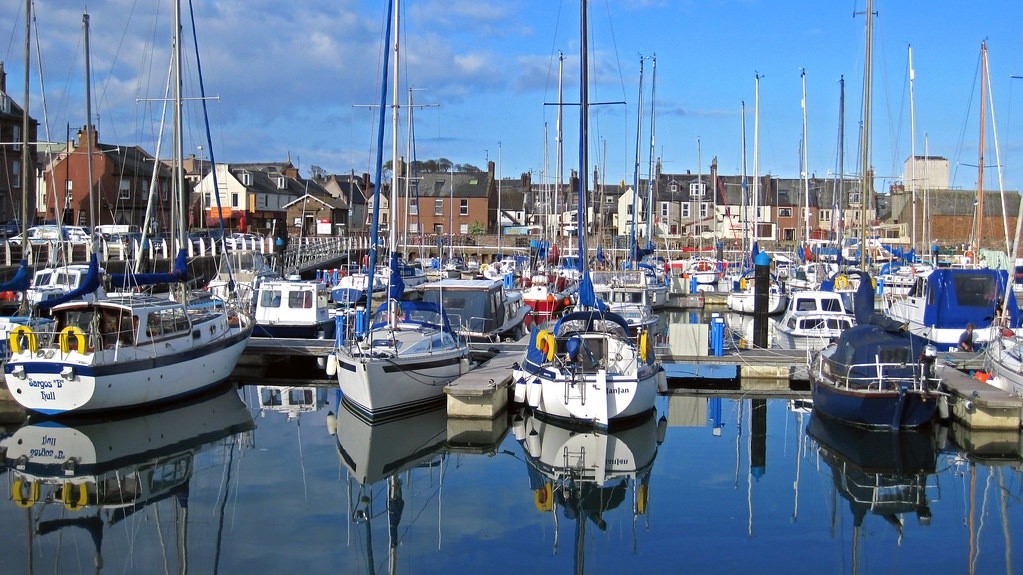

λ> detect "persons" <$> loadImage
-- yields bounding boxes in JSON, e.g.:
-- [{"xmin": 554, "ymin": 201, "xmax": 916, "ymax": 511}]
[
  {"xmin": 957, "ymin": 323, "xmax": 975, "ymax": 352},
  {"xmin": 991, "ymin": 301, "xmax": 1008, "ymax": 330}
]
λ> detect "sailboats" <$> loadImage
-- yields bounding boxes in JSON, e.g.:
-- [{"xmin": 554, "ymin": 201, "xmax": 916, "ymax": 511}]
[
  {"xmin": 325, "ymin": 397, "xmax": 449, "ymax": 575},
  {"xmin": 0, "ymin": 0, "xmax": 1023, "ymax": 432},
  {"xmin": 805, "ymin": 407, "xmax": 947, "ymax": 575},
  {"xmin": 510, "ymin": 406, "xmax": 668, "ymax": 575},
  {"xmin": 0, "ymin": 378, "xmax": 258, "ymax": 575}
]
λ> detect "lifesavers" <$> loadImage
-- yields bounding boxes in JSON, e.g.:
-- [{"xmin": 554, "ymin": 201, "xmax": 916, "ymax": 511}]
[
  {"xmin": 58, "ymin": 325, "xmax": 88, "ymax": 356},
  {"xmin": 9, "ymin": 325, "xmax": 38, "ymax": 352},
  {"xmin": 536, "ymin": 329, "xmax": 558, "ymax": 361},
  {"xmin": 740, "ymin": 278, "xmax": 746, "ymax": 288},
  {"xmin": 12, "ymin": 480, "xmax": 40, "ymax": 508},
  {"xmin": 638, "ymin": 484, "xmax": 648, "ymax": 514},
  {"xmin": 61, "ymin": 482, "xmax": 88, "ymax": 511},
  {"xmin": 533, "ymin": 482, "xmax": 554, "ymax": 511},
  {"xmin": 640, "ymin": 329, "xmax": 650, "ymax": 362},
  {"xmin": 835, "ymin": 276, "xmax": 847, "ymax": 288}
]
